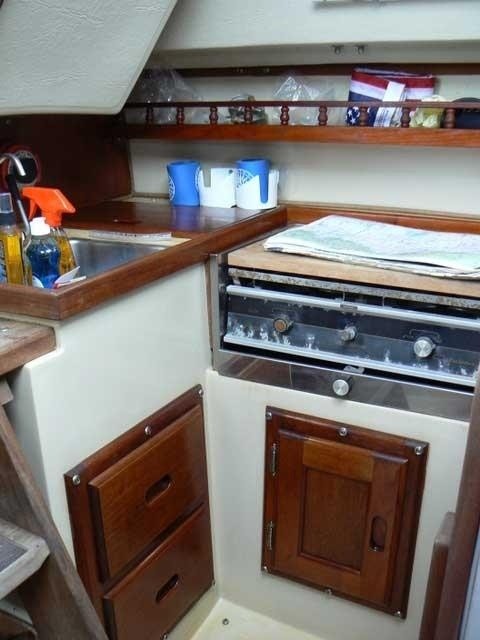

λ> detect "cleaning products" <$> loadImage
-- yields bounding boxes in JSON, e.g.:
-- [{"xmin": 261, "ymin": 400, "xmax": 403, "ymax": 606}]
[
  {"xmin": 0, "ymin": 193, "xmax": 26, "ymax": 286},
  {"xmin": 21, "ymin": 187, "xmax": 81, "ymax": 280}
]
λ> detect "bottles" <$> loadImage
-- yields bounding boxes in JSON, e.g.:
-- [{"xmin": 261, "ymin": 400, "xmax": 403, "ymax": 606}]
[
  {"xmin": 1, "ymin": 189, "xmax": 35, "ymax": 286},
  {"xmin": 22, "ymin": 213, "xmax": 61, "ymax": 289}
]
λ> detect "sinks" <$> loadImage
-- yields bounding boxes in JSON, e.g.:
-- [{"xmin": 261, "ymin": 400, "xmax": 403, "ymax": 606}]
[{"xmin": 1, "ymin": 237, "xmax": 170, "ymax": 289}]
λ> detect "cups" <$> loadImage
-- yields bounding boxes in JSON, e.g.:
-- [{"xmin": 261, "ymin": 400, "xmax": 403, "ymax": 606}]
[
  {"xmin": 165, "ymin": 158, "xmax": 202, "ymax": 207},
  {"xmin": 235, "ymin": 158, "xmax": 269, "ymax": 203}
]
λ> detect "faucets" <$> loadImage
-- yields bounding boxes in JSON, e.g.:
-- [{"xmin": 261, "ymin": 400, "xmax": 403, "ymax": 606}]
[{"xmin": 0, "ymin": 153, "xmax": 26, "ymax": 176}]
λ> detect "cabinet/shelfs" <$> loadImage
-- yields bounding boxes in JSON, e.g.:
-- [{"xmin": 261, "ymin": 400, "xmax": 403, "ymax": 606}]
[
  {"xmin": 63, "ymin": 383, "xmax": 216, "ymax": 640},
  {"xmin": 260, "ymin": 405, "xmax": 429, "ymax": 620}
]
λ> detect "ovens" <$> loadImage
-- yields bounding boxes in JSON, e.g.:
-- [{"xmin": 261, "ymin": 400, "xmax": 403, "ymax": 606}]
[{"xmin": 197, "ymin": 285, "xmax": 477, "ymax": 640}]
